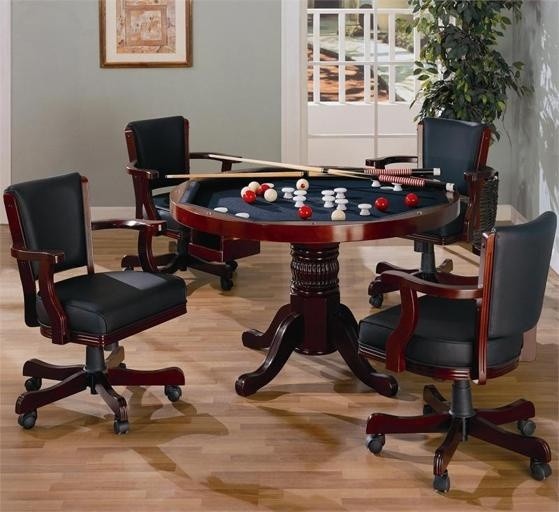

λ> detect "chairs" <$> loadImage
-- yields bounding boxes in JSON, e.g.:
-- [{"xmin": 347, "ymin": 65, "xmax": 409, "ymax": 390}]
[
  {"xmin": 366, "ymin": 117, "xmax": 489, "ymax": 306},
  {"xmin": 358, "ymin": 211, "xmax": 556, "ymax": 493},
  {"xmin": 3, "ymin": 173, "xmax": 186, "ymax": 434},
  {"xmin": 122, "ymin": 116, "xmax": 260, "ymax": 289}
]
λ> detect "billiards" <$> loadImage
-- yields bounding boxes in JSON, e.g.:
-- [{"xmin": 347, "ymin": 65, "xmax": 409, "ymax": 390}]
[{"xmin": 240, "ymin": 177, "xmax": 418, "ymax": 221}]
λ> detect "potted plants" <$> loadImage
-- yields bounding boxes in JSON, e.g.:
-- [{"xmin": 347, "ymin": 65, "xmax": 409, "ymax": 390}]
[{"xmin": 405, "ymin": 0, "xmax": 533, "ymax": 243}]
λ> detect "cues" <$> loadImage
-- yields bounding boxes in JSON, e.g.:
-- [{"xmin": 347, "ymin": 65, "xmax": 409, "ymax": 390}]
[{"xmin": 166, "ymin": 155, "xmax": 458, "ymax": 193}]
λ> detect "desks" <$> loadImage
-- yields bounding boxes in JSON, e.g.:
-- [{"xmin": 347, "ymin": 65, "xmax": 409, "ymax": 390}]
[{"xmin": 169, "ymin": 166, "xmax": 459, "ymax": 396}]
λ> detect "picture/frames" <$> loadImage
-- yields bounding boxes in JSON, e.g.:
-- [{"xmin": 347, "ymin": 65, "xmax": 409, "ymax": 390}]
[{"xmin": 99, "ymin": 0, "xmax": 193, "ymax": 68}]
